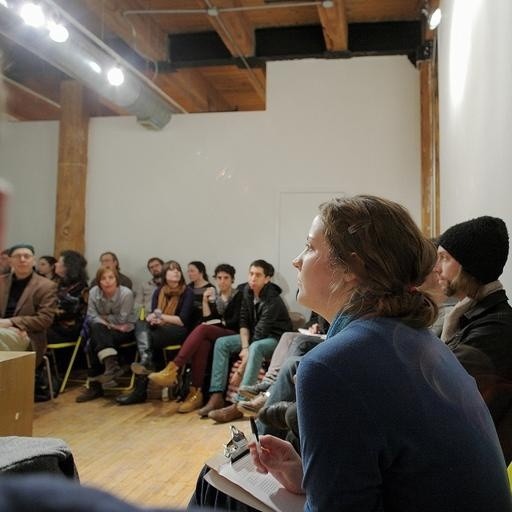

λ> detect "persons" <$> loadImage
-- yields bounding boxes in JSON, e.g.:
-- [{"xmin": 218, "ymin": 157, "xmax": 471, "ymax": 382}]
[
  {"xmin": 256, "ymin": 216, "xmax": 512, "ymax": 470},
  {"xmin": 36, "ymin": 250, "xmax": 89, "ymax": 398},
  {"xmin": 89, "ymin": 253, "xmax": 132, "ymax": 388},
  {"xmin": 76, "ymin": 265, "xmax": 133, "ymax": 402},
  {"xmin": 194, "ymin": 259, "xmax": 292, "ymax": 422},
  {"xmin": 0, "ymin": 245, "xmax": 57, "ymax": 375},
  {"xmin": 0, "ymin": 249, "xmax": 13, "ymax": 274},
  {"xmin": 254, "ymin": 234, "xmax": 459, "ymax": 441},
  {"xmin": 184, "ymin": 262, "xmax": 217, "ymax": 324},
  {"xmin": 116, "ymin": 260, "xmax": 193, "ymax": 403},
  {"xmin": 189, "ymin": 195, "xmax": 511, "ymax": 512},
  {"xmin": 147, "ymin": 264, "xmax": 242, "ymax": 413},
  {"xmin": 39, "ymin": 256, "xmax": 57, "ymax": 282},
  {"xmin": 235, "ymin": 336, "xmax": 324, "ymax": 415},
  {"xmin": 135, "ymin": 257, "xmax": 164, "ymax": 322},
  {"xmin": 239, "ymin": 310, "xmax": 320, "ymax": 399}
]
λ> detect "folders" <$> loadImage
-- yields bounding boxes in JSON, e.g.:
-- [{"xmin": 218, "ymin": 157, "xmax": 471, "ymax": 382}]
[{"xmin": 204, "ymin": 425, "xmax": 307, "ymax": 512}]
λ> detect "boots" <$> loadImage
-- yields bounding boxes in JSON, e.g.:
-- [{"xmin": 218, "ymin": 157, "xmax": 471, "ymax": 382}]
[
  {"xmin": 115, "ymin": 374, "xmax": 149, "ymax": 406},
  {"xmin": 129, "ymin": 327, "xmax": 153, "ymax": 376}
]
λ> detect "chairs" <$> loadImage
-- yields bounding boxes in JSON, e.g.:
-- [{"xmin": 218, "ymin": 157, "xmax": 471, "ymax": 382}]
[
  {"xmin": 159, "ymin": 343, "xmax": 185, "ymax": 391},
  {"xmin": 47, "ymin": 316, "xmax": 92, "ymax": 393},
  {"xmin": 103, "ymin": 304, "xmax": 146, "ymax": 390}
]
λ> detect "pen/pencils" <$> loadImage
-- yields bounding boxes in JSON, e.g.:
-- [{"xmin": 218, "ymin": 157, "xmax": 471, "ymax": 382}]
[{"xmin": 247, "ymin": 414, "xmax": 266, "ymax": 457}]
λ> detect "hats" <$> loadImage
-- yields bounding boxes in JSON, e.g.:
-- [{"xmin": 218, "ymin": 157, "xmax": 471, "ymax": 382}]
[
  {"xmin": 6, "ymin": 242, "xmax": 36, "ymax": 257},
  {"xmin": 436, "ymin": 214, "xmax": 510, "ymax": 285}
]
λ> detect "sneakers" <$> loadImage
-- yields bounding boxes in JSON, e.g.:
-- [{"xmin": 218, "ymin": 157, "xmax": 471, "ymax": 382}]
[
  {"xmin": 206, "ymin": 396, "xmax": 245, "ymax": 425},
  {"xmin": 147, "ymin": 361, "xmax": 179, "ymax": 387},
  {"xmin": 237, "ymin": 391, "xmax": 270, "ymax": 414},
  {"xmin": 256, "ymin": 401, "xmax": 294, "ymax": 430},
  {"xmin": 178, "ymin": 385, "xmax": 204, "ymax": 416},
  {"xmin": 193, "ymin": 392, "xmax": 227, "ymax": 417},
  {"xmin": 237, "ymin": 387, "xmax": 262, "ymax": 399},
  {"xmin": 74, "ymin": 377, "xmax": 105, "ymax": 408},
  {"xmin": 97, "ymin": 353, "xmax": 123, "ymax": 386}
]
